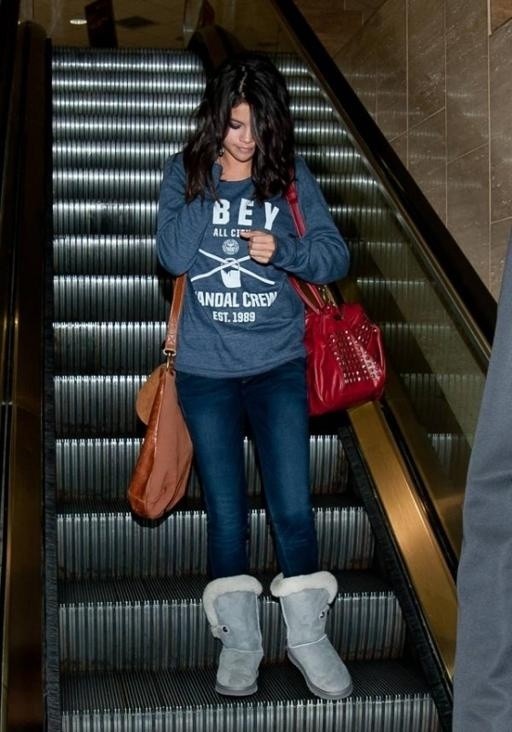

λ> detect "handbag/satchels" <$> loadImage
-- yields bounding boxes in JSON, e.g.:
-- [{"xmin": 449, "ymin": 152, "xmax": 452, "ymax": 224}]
[
  {"xmin": 285, "ymin": 269, "xmax": 388, "ymax": 419},
  {"xmin": 126, "ymin": 360, "xmax": 194, "ymax": 522}
]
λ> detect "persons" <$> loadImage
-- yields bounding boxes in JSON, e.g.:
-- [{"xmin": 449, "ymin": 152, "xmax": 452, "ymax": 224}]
[{"xmin": 157, "ymin": 48, "xmax": 354, "ymax": 700}]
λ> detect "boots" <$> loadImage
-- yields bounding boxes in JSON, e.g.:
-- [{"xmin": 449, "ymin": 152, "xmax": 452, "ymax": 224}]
[
  {"xmin": 269, "ymin": 569, "xmax": 355, "ymax": 701},
  {"xmin": 200, "ymin": 572, "xmax": 265, "ymax": 699}
]
